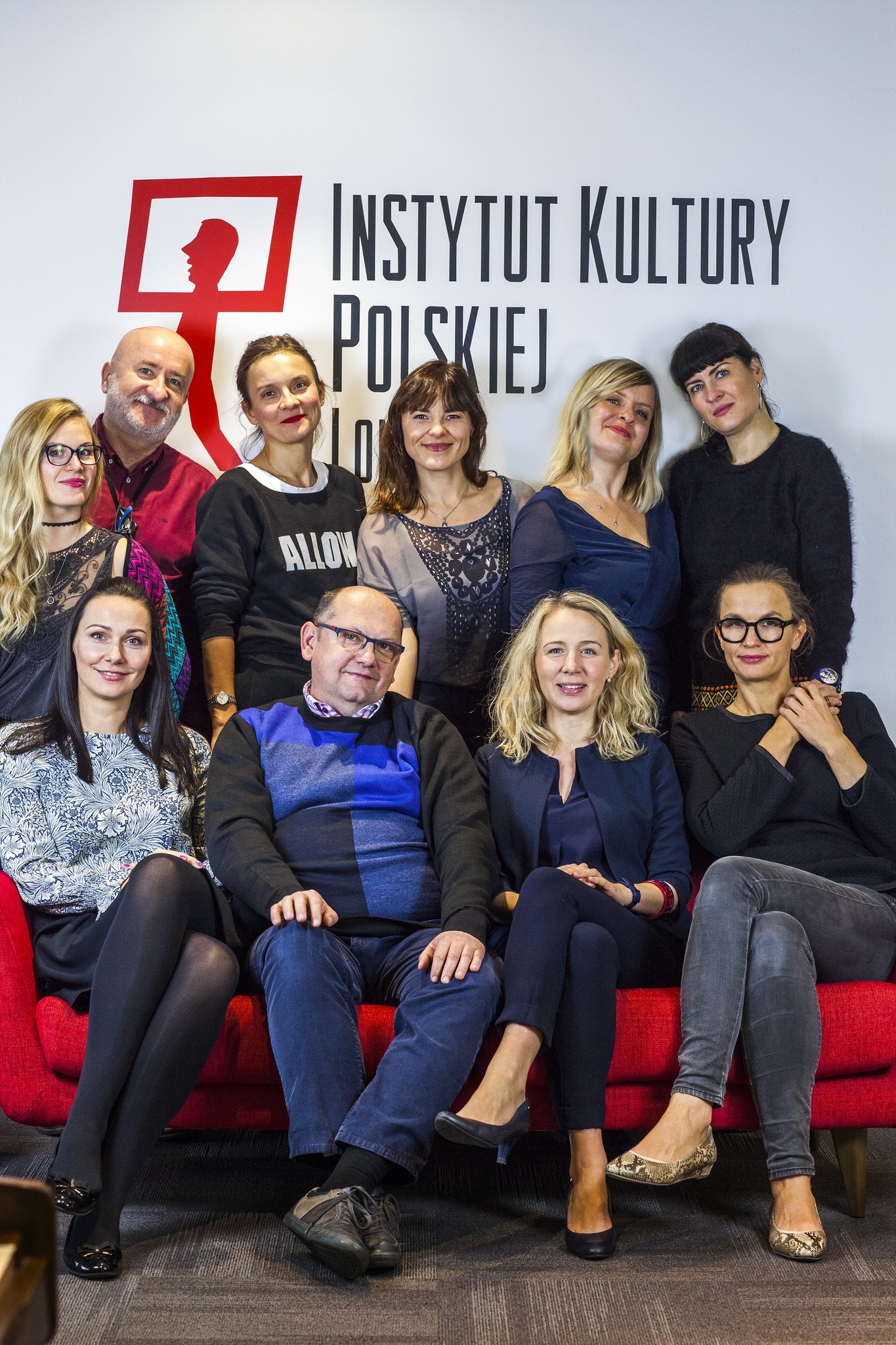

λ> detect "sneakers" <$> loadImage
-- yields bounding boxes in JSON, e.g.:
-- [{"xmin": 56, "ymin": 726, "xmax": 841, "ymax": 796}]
[
  {"xmin": 282, "ymin": 1186, "xmax": 380, "ymax": 1280},
  {"xmin": 355, "ymin": 1187, "xmax": 403, "ymax": 1271}
]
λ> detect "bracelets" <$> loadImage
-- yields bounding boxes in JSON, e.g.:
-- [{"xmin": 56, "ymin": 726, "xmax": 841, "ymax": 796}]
[
  {"xmin": 638, "ymin": 879, "xmax": 675, "ymax": 920},
  {"xmin": 615, "ymin": 876, "xmax": 640, "ymax": 911}
]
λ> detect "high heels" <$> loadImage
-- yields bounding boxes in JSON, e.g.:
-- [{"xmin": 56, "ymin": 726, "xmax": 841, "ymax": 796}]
[
  {"xmin": 436, "ymin": 1098, "xmax": 532, "ymax": 1167},
  {"xmin": 606, "ymin": 1124, "xmax": 717, "ymax": 1185},
  {"xmin": 770, "ymin": 1195, "xmax": 827, "ymax": 1260},
  {"xmin": 565, "ymin": 1180, "xmax": 618, "ymax": 1259}
]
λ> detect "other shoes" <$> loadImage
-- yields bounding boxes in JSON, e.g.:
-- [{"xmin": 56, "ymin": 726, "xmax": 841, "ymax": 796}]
[
  {"xmin": 45, "ymin": 1134, "xmax": 103, "ymax": 1217},
  {"xmin": 64, "ymin": 1216, "xmax": 122, "ymax": 1279}
]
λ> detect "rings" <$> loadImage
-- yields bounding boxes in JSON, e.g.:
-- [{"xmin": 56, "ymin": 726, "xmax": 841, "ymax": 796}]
[{"xmin": 824, "ymin": 696, "xmax": 833, "ymax": 708}]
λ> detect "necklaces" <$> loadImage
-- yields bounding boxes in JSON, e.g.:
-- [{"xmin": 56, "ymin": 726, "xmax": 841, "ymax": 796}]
[
  {"xmin": 262, "ymin": 447, "xmax": 291, "ymax": 487},
  {"xmin": 39, "ymin": 525, "xmax": 81, "ymax": 605},
  {"xmin": 571, "ymin": 467, "xmax": 628, "ymax": 527},
  {"xmin": 41, "ymin": 516, "xmax": 82, "ymax": 528},
  {"xmin": 420, "ymin": 479, "xmax": 472, "ymax": 528}
]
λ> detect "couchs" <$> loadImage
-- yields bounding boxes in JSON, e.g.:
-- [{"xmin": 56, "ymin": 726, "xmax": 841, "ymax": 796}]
[{"xmin": 1, "ymin": 869, "xmax": 896, "ymax": 1218}]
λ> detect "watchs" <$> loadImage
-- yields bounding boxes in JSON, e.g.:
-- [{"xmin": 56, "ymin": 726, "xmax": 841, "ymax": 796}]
[
  {"xmin": 806, "ymin": 665, "xmax": 843, "ymax": 694},
  {"xmin": 205, "ymin": 691, "xmax": 237, "ymax": 707}
]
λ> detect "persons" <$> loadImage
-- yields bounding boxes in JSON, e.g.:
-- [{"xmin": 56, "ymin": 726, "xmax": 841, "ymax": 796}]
[
  {"xmin": 0, "ymin": 397, "xmax": 196, "ymax": 726},
  {"xmin": 204, "ymin": 583, "xmax": 501, "ymax": 1285},
  {"xmin": 2, "ymin": 573, "xmax": 248, "ymax": 1281},
  {"xmin": 510, "ymin": 358, "xmax": 689, "ymax": 747},
  {"xmin": 357, "ymin": 361, "xmax": 538, "ymax": 760},
  {"xmin": 79, "ymin": 326, "xmax": 218, "ymax": 601},
  {"xmin": 190, "ymin": 330, "xmax": 368, "ymax": 749},
  {"xmin": 602, "ymin": 561, "xmax": 895, "ymax": 1261},
  {"xmin": 656, "ymin": 321, "xmax": 856, "ymax": 729},
  {"xmin": 432, "ymin": 588, "xmax": 695, "ymax": 1262}
]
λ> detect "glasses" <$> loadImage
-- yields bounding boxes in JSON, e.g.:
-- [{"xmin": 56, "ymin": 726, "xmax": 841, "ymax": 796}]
[
  {"xmin": 714, "ymin": 617, "xmax": 798, "ymax": 643},
  {"xmin": 315, "ymin": 622, "xmax": 405, "ymax": 664},
  {"xmin": 41, "ymin": 444, "xmax": 103, "ymax": 465}
]
